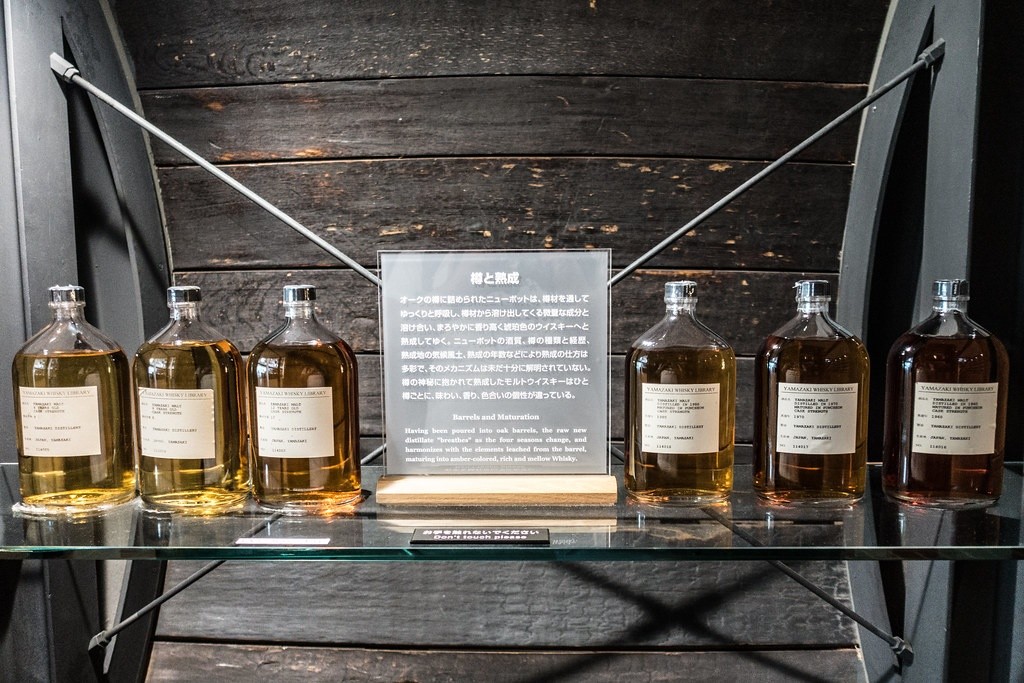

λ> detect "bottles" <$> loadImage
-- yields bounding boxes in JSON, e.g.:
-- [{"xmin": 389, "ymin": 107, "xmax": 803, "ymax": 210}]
[
  {"xmin": 131, "ymin": 287, "xmax": 245, "ymax": 513},
  {"xmin": 753, "ymin": 280, "xmax": 870, "ymax": 507},
  {"xmin": 246, "ymin": 285, "xmax": 362, "ymax": 514},
  {"xmin": 882, "ymin": 280, "xmax": 1009, "ymax": 511},
  {"xmin": 11, "ymin": 286, "xmax": 130, "ymax": 514},
  {"xmin": 624, "ymin": 281, "xmax": 737, "ymax": 507}
]
